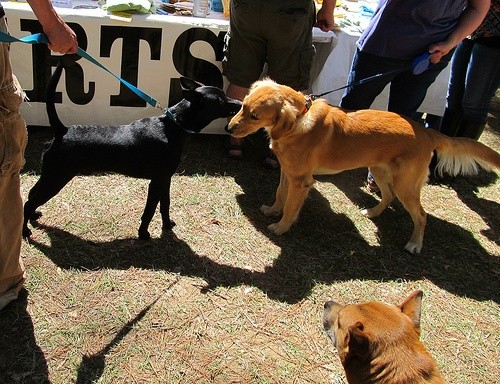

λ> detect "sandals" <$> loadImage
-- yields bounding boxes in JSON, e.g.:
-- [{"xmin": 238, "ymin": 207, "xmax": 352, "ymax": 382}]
[
  {"xmin": 228, "ymin": 141, "xmax": 243, "ymax": 156},
  {"xmin": 264, "ymin": 153, "xmax": 281, "ymax": 169}
]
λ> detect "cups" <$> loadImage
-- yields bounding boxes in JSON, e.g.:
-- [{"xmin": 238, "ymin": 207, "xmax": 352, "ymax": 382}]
[{"xmin": 192, "ymin": 0, "xmax": 212, "ymax": 18}]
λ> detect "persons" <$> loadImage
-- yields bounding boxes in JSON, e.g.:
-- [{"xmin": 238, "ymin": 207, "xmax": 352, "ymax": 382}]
[
  {"xmin": 222, "ymin": 0, "xmax": 337, "ymax": 170},
  {"xmin": 429, "ymin": 0, "xmax": 500, "ymax": 183},
  {"xmin": 0, "ymin": 0, "xmax": 79, "ymax": 310},
  {"xmin": 341, "ymin": 0, "xmax": 491, "ymax": 188}
]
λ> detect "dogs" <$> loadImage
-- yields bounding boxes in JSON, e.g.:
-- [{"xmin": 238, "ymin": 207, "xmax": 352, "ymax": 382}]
[
  {"xmin": 22, "ymin": 59, "xmax": 244, "ymax": 246},
  {"xmin": 323, "ymin": 290, "xmax": 449, "ymax": 384},
  {"xmin": 224, "ymin": 72, "xmax": 500, "ymax": 257}
]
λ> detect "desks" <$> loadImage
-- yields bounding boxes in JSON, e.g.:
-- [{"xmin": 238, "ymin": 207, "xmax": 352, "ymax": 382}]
[{"xmin": 0, "ymin": 0, "xmax": 457, "ymax": 134}]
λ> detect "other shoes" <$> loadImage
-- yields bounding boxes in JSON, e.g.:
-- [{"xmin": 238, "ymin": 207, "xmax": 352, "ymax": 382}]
[
  {"xmin": 367, "ymin": 172, "xmax": 380, "ymax": 193},
  {"xmin": 430, "ymin": 166, "xmax": 456, "ymax": 185}
]
